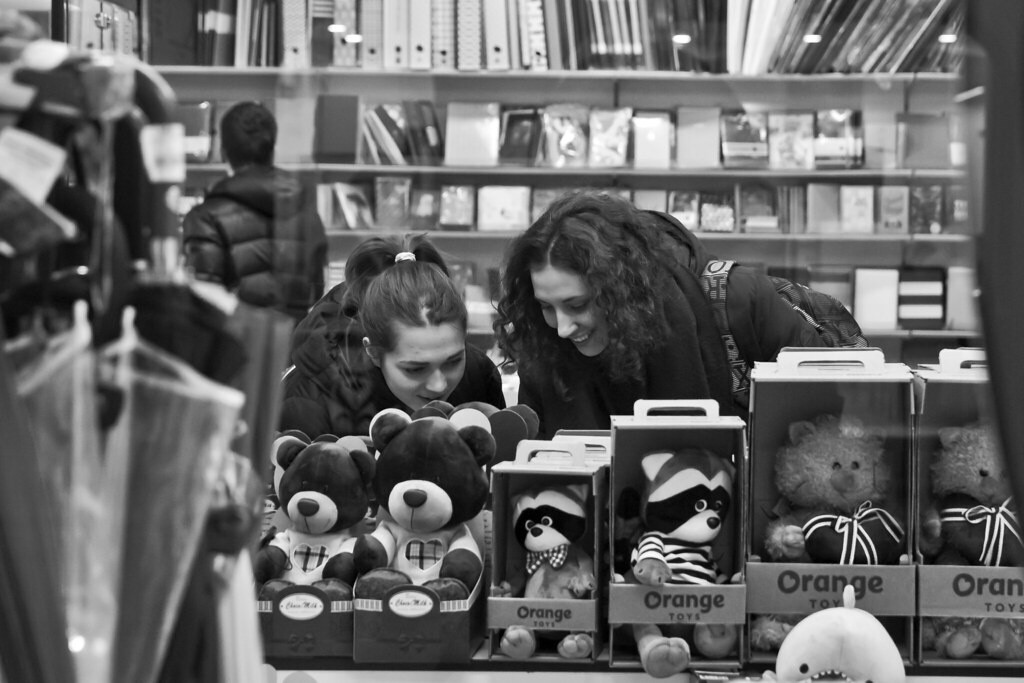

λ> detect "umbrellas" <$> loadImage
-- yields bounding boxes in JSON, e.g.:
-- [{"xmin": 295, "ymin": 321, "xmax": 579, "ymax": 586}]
[{"xmin": 0, "ymin": 62, "xmax": 248, "ymax": 683}]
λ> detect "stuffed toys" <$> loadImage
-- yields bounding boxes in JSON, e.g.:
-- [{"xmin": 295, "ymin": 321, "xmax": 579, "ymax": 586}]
[{"xmin": 253, "ymin": 400, "xmax": 1024, "ymax": 683}]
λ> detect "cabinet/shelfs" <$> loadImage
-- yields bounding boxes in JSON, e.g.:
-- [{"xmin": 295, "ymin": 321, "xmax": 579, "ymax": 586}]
[{"xmin": 148, "ymin": 63, "xmax": 984, "ymax": 340}]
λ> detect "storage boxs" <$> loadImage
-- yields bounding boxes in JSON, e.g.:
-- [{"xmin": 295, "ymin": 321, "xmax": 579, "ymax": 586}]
[
  {"xmin": 485, "ymin": 438, "xmax": 609, "ymax": 664},
  {"xmin": 910, "ymin": 347, "xmax": 1024, "ymax": 669},
  {"xmin": 745, "ymin": 351, "xmax": 918, "ymax": 666},
  {"xmin": 607, "ymin": 399, "xmax": 749, "ymax": 666},
  {"xmin": 255, "ymin": 585, "xmax": 351, "ymax": 659},
  {"xmin": 352, "ymin": 549, "xmax": 487, "ymax": 664}
]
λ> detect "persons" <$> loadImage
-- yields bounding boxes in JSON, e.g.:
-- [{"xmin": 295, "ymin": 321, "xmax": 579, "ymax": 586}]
[
  {"xmin": 275, "ymin": 232, "xmax": 506, "ymax": 439},
  {"xmin": 493, "ymin": 193, "xmax": 826, "ymax": 439},
  {"xmin": 182, "ymin": 99, "xmax": 328, "ymax": 325}
]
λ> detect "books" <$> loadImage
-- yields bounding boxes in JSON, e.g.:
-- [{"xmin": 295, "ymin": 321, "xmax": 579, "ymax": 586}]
[
  {"xmin": 136, "ymin": 0, "xmax": 972, "ymax": 74},
  {"xmin": 314, "ymin": 95, "xmax": 974, "ymax": 330}
]
